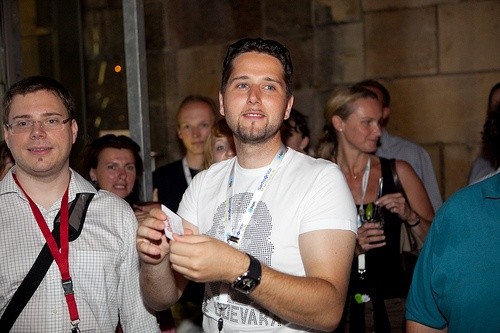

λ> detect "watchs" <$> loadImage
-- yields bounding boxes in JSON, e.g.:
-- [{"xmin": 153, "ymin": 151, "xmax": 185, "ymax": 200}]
[{"xmin": 230, "ymin": 253, "xmax": 261, "ymax": 294}]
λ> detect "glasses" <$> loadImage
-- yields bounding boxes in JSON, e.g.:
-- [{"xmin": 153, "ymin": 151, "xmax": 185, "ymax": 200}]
[{"xmin": 4, "ymin": 116, "xmax": 70, "ymax": 135}]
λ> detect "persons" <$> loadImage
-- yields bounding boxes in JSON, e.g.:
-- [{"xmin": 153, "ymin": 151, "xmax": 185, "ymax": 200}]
[
  {"xmin": 136, "ymin": 37, "xmax": 359, "ymax": 333},
  {"xmin": 280, "ymin": 79, "xmax": 444, "ymax": 215},
  {"xmin": 467, "ymin": 83, "xmax": 500, "ymax": 183},
  {"xmin": 0, "ymin": 74, "xmax": 161, "ymax": 333},
  {"xmin": 313, "ymin": 86, "xmax": 435, "ymax": 333},
  {"xmin": 405, "ymin": 99, "xmax": 500, "ymax": 333},
  {"xmin": 0, "ymin": 95, "xmax": 237, "ymax": 332}
]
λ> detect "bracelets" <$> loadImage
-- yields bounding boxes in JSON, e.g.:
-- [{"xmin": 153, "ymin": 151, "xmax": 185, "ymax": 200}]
[{"xmin": 408, "ymin": 211, "xmax": 420, "ymax": 227}]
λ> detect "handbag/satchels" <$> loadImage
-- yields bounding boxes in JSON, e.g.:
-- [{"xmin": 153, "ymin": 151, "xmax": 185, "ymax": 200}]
[{"xmin": 399, "ymin": 222, "xmax": 424, "ymax": 275}]
